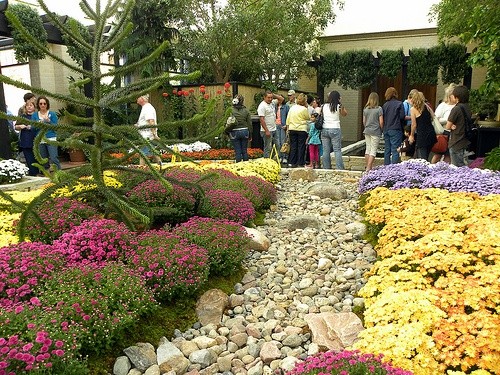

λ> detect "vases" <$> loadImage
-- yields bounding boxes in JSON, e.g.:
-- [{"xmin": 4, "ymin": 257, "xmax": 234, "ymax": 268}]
[{"xmin": 69, "ymin": 148, "xmax": 85, "ymax": 162}]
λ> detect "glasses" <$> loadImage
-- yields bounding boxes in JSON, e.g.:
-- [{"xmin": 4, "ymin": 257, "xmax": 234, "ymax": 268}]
[
  {"xmin": 39, "ymin": 102, "xmax": 46, "ymax": 105},
  {"xmin": 288, "ymin": 95, "xmax": 292, "ymax": 96}
]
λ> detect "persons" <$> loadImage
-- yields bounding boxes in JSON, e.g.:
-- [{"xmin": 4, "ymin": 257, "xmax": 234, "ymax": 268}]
[
  {"xmin": 403, "ymin": 89, "xmax": 437, "ymax": 162},
  {"xmin": 382, "ymin": 87, "xmax": 412, "ymax": 165},
  {"xmin": 397, "ymin": 126, "xmax": 412, "ymax": 163},
  {"xmin": 431, "ymin": 83, "xmax": 472, "ymax": 167},
  {"xmin": 257, "ymin": 90, "xmax": 284, "ymax": 163},
  {"xmin": 363, "ymin": 92, "xmax": 383, "ymax": 172},
  {"xmin": 225, "ymin": 94, "xmax": 253, "ymax": 163},
  {"xmin": 6, "ymin": 104, "xmax": 13, "ymax": 130},
  {"xmin": 136, "ymin": 90, "xmax": 162, "ymax": 166},
  {"xmin": 15, "ymin": 93, "xmax": 61, "ymax": 177},
  {"xmin": 320, "ymin": 90, "xmax": 347, "ymax": 170},
  {"xmin": 275, "ymin": 89, "xmax": 321, "ymax": 169}
]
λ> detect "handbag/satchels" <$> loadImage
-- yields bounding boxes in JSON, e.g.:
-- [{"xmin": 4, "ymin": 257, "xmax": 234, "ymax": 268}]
[
  {"xmin": 315, "ymin": 104, "xmax": 324, "ymax": 130},
  {"xmin": 432, "ymin": 133, "xmax": 448, "ymax": 154},
  {"xmin": 432, "ymin": 118, "xmax": 444, "ymax": 135},
  {"xmin": 280, "ymin": 133, "xmax": 290, "ymax": 154},
  {"xmin": 224, "ymin": 112, "xmax": 239, "ymax": 135},
  {"xmin": 465, "ymin": 118, "xmax": 475, "ymax": 141}
]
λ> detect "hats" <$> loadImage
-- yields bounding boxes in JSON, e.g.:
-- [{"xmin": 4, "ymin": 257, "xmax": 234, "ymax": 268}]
[{"xmin": 287, "ymin": 89, "xmax": 295, "ymax": 95}]
